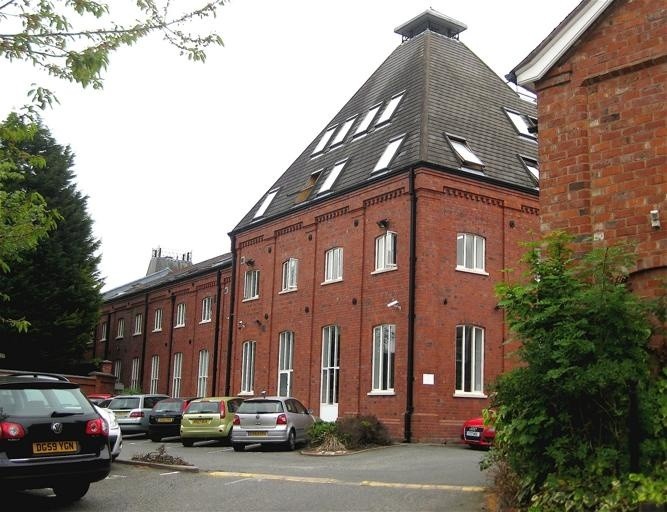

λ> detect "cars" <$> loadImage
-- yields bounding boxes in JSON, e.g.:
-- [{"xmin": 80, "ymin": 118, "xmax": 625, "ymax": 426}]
[
  {"xmin": 148, "ymin": 397, "xmax": 205, "ymax": 442},
  {"xmin": 231, "ymin": 396, "xmax": 317, "ymax": 452},
  {"xmin": 84, "ymin": 394, "xmax": 123, "ymax": 460},
  {"xmin": 462, "ymin": 413, "xmax": 498, "ymax": 450},
  {"xmin": 180, "ymin": 396, "xmax": 249, "ymax": 447},
  {"xmin": 107, "ymin": 393, "xmax": 171, "ymax": 435}
]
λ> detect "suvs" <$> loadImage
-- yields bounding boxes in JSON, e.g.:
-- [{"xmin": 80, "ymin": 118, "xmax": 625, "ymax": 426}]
[{"xmin": 0, "ymin": 369, "xmax": 112, "ymax": 502}]
